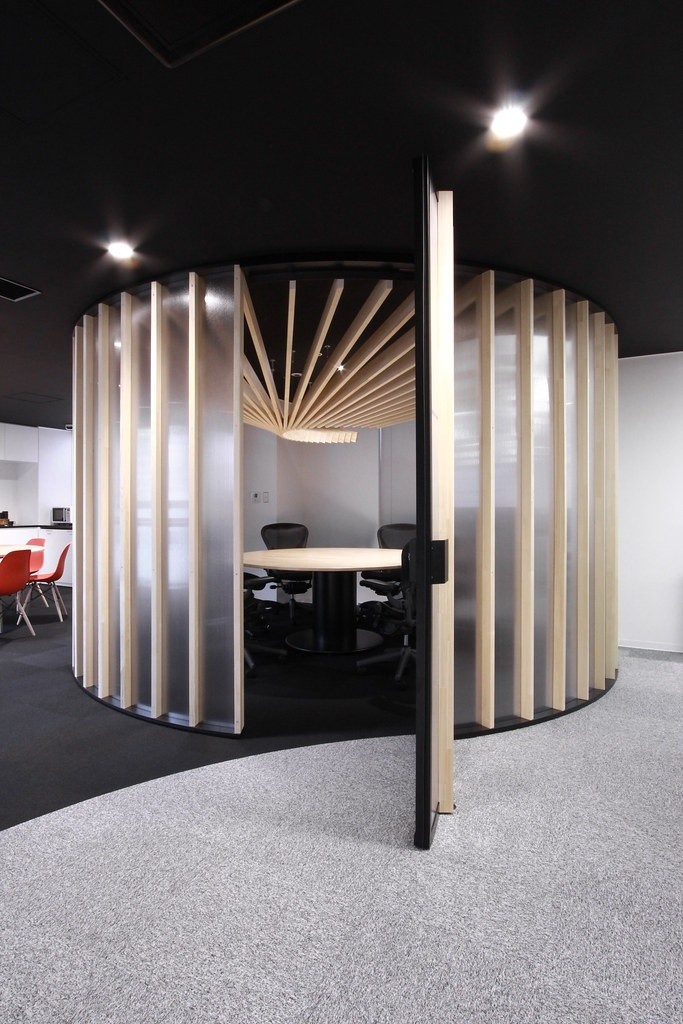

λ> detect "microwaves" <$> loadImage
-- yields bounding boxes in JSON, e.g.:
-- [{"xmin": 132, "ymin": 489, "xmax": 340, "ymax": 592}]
[{"xmin": 50, "ymin": 507, "xmax": 71, "ymax": 524}]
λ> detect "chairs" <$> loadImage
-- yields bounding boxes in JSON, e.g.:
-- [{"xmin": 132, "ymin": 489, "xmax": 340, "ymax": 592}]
[
  {"xmin": 243, "ymin": 571, "xmax": 270, "ymax": 678},
  {"xmin": 358, "ymin": 521, "xmax": 416, "ymax": 598},
  {"xmin": 0, "ymin": 538, "xmax": 71, "ymax": 636},
  {"xmin": 355, "ymin": 539, "xmax": 419, "ymax": 682},
  {"xmin": 260, "ymin": 522, "xmax": 312, "ymax": 632}
]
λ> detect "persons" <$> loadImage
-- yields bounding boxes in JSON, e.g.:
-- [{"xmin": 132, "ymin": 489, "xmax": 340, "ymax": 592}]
[{"xmin": 0, "ymin": 513, "xmax": 7, "ymax": 527}]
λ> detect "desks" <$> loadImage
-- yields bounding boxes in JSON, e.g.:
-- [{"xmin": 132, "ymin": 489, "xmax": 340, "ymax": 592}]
[
  {"xmin": 0, "ymin": 544, "xmax": 43, "ymax": 615},
  {"xmin": 240, "ymin": 547, "xmax": 403, "ymax": 654}
]
